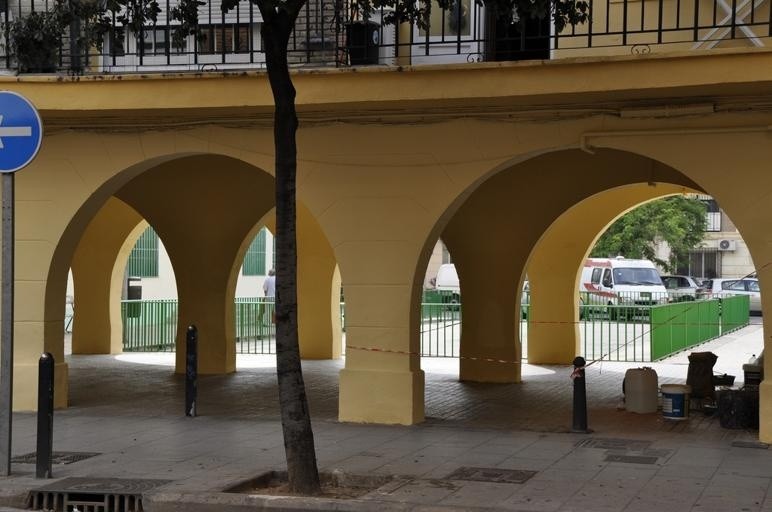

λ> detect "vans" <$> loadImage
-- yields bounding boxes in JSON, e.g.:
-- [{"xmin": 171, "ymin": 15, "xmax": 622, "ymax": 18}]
[
  {"xmin": 579, "ymin": 254, "xmax": 669, "ymax": 320},
  {"xmin": 434, "ymin": 264, "xmax": 461, "ymax": 310},
  {"xmin": 698, "ymin": 277, "xmax": 763, "ymax": 315}
]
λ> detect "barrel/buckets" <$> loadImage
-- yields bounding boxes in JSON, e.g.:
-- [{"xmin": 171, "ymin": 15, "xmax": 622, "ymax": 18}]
[
  {"xmin": 748, "ymin": 353, "xmax": 758, "ymax": 364},
  {"xmin": 712, "ymin": 389, "xmax": 759, "ymax": 430},
  {"xmin": 625, "ymin": 367, "xmax": 657, "ymax": 413},
  {"xmin": 661, "ymin": 384, "xmax": 692, "ymax": 420}
]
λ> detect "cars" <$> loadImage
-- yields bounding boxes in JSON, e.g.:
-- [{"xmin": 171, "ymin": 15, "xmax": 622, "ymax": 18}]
[{"xmin": 518, "ymin": 281, "xmax": 531, "ymax": 318}]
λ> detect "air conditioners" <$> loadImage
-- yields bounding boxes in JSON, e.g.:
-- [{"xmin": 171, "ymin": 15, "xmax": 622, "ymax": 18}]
[{"xmin": 717, "ymin": 237, "xmax": 736, "ymax": 253}]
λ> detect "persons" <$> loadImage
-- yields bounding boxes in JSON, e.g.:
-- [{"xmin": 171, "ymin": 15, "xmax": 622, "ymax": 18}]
[{"xmin": 263, "ymin": 269, "xmax": 276, "ymax": 326}]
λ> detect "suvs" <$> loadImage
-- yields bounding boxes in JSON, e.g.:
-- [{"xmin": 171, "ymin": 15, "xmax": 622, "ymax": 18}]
[{"xmin": 657, "ymin": 274, "xmax": 700, "ymax": 305}]
